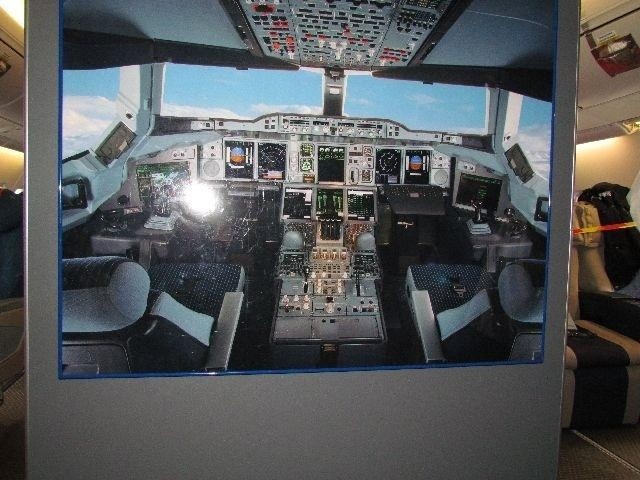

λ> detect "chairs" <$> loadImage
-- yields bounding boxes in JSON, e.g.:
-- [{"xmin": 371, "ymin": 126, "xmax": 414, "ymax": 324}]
[
  {"xmin": 404, "ymin": 259, "xmax": 546, "ymax": 365},
  {"xmin": 61, "ymin": 256, "xmax": 245, "ymax": 374},
  {"xmin": 0, "ymin": 188, "xmax": 26, "ymax": 426},
  {"xmin": 576, "ymin": 199, "xmax": 640, "ymax": 299},
  {"xmin": 561, "ymin": 201, "xmax": 640, "ymax": 430}
]
